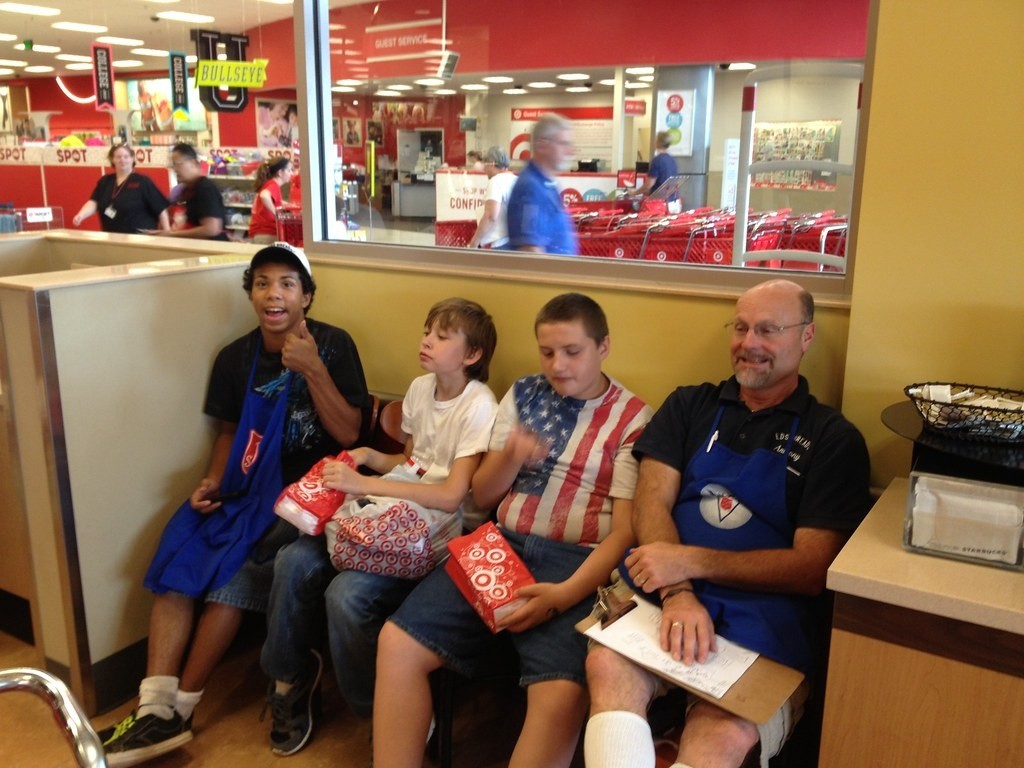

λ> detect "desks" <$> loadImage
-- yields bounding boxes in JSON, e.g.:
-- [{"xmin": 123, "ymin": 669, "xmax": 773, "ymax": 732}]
[{"xmin": 825, "ymin": 475, "xmax": 1024, "ymax": 768}]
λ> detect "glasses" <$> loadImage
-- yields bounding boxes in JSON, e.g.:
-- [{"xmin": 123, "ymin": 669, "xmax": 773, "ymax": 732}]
[{"xmin": 725, "ymin": 322, "xmax": 805, "ymax": 337}]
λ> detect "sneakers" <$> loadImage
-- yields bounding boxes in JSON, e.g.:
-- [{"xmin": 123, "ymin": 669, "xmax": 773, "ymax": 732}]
[
  {"xmin": 259, "ymin": 649, "xmax": 323, "ymax": 756},
  {"xmin": 97, "ymin": 709, "xmax": 194, "ymax": 768}
]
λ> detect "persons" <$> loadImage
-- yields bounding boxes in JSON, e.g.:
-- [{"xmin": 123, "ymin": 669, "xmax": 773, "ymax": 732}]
[
  {"xmin": 73, "ymin": 145, "xmax": 169, "ymax": 233},
  {"xmin": 469, "ymin": 146, "xmax": 518, "ymax": 252},
  {"xmin": 96, "ymin": 241, "xmax": 370, "ymax": 768},
  {"xmin": 508, "ymin": 112, "xmax": 580, "ymax": 258},
  {"xmin": 627, "ymin": 132, "xmax": 683, "ymax": 215},
  {"xmin": 373, "ymin": 294, "xmax": 649, "ymax": 768},
  {"xmin": 584, "ymin": 281, "xmax": 872, "ymax": 768},
  {"xmin": 258, "ymin": 297, "xmax": 497, "ymax": 758},
  {"xmin": 469, "ymin": 151, "xmax": 484, "ymax": 170},
  {"xmin": 141, "ymin": 144, "xmax": 230, "ymax": 242},
  {"xmin": 249, "ymin": 158, "xmax": 295, "ymax": 244}
]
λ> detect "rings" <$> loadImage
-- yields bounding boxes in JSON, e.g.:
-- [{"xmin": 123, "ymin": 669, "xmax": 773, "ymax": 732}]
[{"xmin": 671, "ymin": 622, "xmax": 684, "ymax": 629}]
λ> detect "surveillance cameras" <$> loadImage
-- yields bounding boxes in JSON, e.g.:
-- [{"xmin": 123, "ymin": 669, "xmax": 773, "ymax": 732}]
[
  {"xmin": 585, "ymin": 82, "xmax": 593, "ymax": 88},
  {"xmin": 515, "ymin": 85, "xmax": 522, "ymax": 88},
  {"xmin": 719, "ymin": 64, "xmax": 730, "ymax": 69}
]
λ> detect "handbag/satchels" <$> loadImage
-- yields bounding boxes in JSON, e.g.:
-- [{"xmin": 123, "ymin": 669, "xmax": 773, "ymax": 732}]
[
  {"xmin": 445, "ymin": 520, "xmax": 536, "ymax": 633},
  {"xmin": 274, "ymin": 451, "xmax": 357, "ymax": 537},
  {"xmin": 324, "ymin": 464, "xmax": 463, "ymax": 579}
]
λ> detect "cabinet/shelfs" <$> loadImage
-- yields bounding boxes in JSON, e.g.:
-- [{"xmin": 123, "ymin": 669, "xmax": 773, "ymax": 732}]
[{"xmin": 208, "ymin": 174, "xmax": 257, "ymax": 231}]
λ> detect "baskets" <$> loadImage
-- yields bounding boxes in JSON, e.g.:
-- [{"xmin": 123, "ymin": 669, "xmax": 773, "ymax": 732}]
[{"xmin": 905, "ymin": 382, "xmax": 1024, "ymax": 444}]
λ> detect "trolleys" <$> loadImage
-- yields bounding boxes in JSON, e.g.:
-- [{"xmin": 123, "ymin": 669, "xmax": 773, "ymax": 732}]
[
  {"xmin": 277, "ymin": 207, "xmax": 303, "ymax": 250},
  {"xmin": 434, "ymin": 209, "xmax": 848, "ymax": 276}
]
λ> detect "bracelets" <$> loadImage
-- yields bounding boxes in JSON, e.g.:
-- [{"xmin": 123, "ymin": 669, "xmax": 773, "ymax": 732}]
[{"xmin": 660, "ymin": 587, "xmax": 695, "ymax": 609}]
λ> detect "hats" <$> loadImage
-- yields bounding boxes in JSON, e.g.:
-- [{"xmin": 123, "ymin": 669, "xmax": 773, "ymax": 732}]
[{"xmin": 252, "ymin": 241, "xmax": 311, "ymax": 280}]
